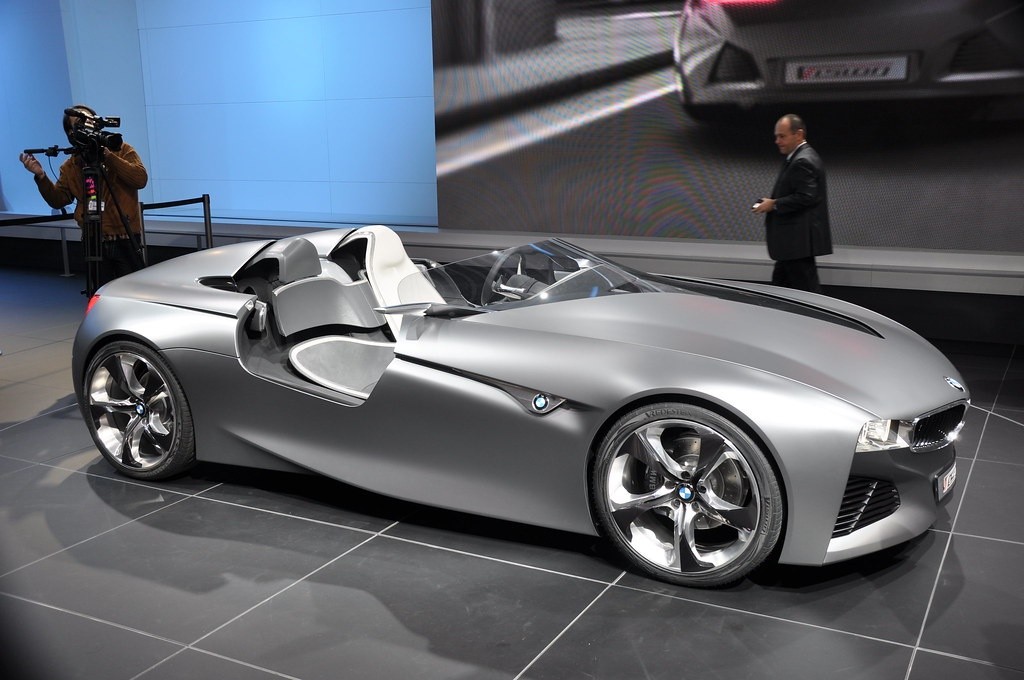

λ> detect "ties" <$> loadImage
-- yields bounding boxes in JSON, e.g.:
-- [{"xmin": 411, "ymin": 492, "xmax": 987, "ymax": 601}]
[{"xmin": 784, "ymin": 159, "xmax": 789, "ymax": 172}]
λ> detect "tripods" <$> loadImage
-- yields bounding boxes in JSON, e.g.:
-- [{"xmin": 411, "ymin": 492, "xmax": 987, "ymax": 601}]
[{"xmin": 72, "ymin": 157, "xmax": 147, "ymax": 302}]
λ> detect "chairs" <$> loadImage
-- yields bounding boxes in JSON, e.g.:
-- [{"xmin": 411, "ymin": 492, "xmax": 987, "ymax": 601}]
[{"xmin": 268, "ymin": 222, "xmax": 451, "ymax": 403}]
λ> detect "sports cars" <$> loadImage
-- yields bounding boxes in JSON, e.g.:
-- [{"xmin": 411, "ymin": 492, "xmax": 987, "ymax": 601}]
[
  {"xmin": 72, "ymin": 224, "xmax": 970, "ymax": 588},
  {"xmin": 673, "ymin": 2, "xmax": 1024, "ymax": 123}
]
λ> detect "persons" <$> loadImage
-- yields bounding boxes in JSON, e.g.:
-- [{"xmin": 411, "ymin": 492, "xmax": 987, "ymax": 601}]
[
  {"xmin": 19, "ymin": 105, "xmax": 148, "ymax": 299},
  {"xmin": 752, "ymin": 115, "xmax": 833, "ymax": 294}
]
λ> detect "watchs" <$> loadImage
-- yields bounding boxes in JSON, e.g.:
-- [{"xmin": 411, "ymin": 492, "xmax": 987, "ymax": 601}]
[{"xmin": 772, "ymin": 203, "xmax": 777, "ymax": 210}]
